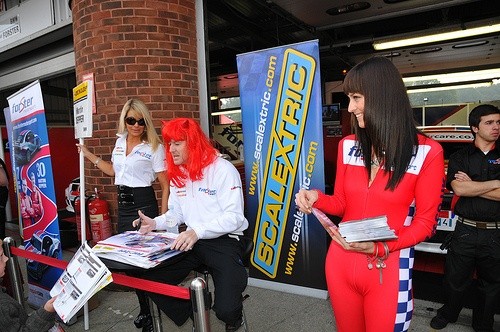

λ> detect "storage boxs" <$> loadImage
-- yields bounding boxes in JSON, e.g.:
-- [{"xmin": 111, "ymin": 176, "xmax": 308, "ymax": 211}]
[{"xmin": 27, "ymin": 282, "xmax": 101, "ymax": 327}]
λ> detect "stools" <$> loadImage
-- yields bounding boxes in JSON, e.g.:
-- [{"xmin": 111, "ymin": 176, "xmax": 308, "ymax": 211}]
[{"xmin": 190, "ymin": 238, "xmax": 255, "ymax": 332}]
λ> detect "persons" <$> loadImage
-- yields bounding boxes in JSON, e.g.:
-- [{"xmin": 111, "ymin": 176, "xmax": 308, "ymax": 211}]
[
  {"xmin": 76, "ymin": 99, "xmax": 170, "ymax": 332},
  {"xmin": 0, "ymin": 158, "xmax": 10, "ymax": 250},
  {"xmin": 294, "ymin": 56, "xmax": 445, "ymax": 332},
  {"xmin": 0, "ymin": 239, "xmax": 58, "ymax": 332},
  {"xmin": 429, "ymin": 104, "xmax": 500, "ymax": 332},
  {"xmin": 132, "ymin": 119, "xmax": 250, "ymax": 332}
]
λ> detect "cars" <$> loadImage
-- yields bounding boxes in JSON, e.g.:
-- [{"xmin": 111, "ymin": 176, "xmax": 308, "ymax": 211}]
[
  {"xmin": 13, "ymin": 129, "xmax": 42, "ymax": 166},
  {"xmin": 64, "ymin": 177, "xmax": 80, "ymax": 214},
  {"xmin": 24, "ymin": 229, "xmax": 60, "ymax": 282}
]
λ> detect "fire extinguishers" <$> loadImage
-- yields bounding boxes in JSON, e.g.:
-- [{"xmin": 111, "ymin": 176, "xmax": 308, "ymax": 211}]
[
  {"xmin": 85, "ymin": 188, "xmax": 113, "ymax": 247},
  {"xmin": 66, "ymin": 177, "xmax": 92, "ymax": 241}
]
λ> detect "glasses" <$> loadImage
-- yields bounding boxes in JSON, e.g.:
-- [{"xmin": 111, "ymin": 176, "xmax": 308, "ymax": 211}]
[{"xmin": 125, "ymin": 117, "xmax": 145, "ymax": 126}]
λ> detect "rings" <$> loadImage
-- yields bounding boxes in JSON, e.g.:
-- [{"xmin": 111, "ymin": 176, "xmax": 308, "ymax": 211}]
[{"xmin": 295, "ymin": 206, "xmax": 300, "ymax": 211}]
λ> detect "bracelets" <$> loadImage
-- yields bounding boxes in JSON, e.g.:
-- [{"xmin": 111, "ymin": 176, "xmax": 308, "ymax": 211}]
[
  {"xmin": 367, "ymin": 240, "xmax": 388, "ymax": 270},
  {"xmin": 94, "ymin": 157, "xmax": 101, "ymax": 166}
]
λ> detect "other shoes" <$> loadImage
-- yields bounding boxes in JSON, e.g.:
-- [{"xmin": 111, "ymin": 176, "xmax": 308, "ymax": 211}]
[
  {"xmin": 225, "ymin": 303, "xmax": 243, "ymax": 332},
  {"xmin": 475, "ymin": 329, "xmax": 490, "ymax": 332},
  {"xmin": 142, "ymin": 320, "xmax": 154, "ymax": 332},
  {"xmin": 190, "ymin": 292, "xmax": 212, "ymax": 332},
  {"xmin": 430, "ymin": 312, "xmax": 458, "ymax": 329},
  {"xmin": 134, "ymin": 313, "xmax": 151, "ymax": 328}
]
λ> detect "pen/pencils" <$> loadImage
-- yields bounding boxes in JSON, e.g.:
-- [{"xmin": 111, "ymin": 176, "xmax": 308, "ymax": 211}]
[
  {"xmin": 148, "ymin": 248, "xmax": 170, "ymax": 258},
  {"xmin": 137, "ymin": 210, "xmax": 145, "ymax": 232},
  {"xmin": 149, "ymin": 249, "xmax": 176, "ymax": 260}
]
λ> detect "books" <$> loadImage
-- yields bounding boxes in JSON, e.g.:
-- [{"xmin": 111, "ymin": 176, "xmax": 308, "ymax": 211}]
[{"xmin": 49, "ymin": 241, "xmax": 114, "ymax": 323}]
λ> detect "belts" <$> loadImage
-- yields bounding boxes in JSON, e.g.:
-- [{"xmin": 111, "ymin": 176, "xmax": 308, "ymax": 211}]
[{"xmin": 458, "ymin": 216, "xmax": 500, "ymax": 229}]
